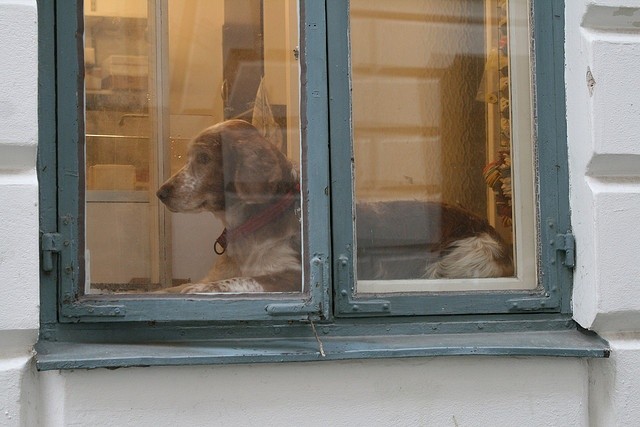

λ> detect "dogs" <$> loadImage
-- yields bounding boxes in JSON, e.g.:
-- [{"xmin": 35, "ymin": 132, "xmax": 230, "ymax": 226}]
[{"xmin": 155, "ymin": 120, "xmax": 516, "ymax": 295}]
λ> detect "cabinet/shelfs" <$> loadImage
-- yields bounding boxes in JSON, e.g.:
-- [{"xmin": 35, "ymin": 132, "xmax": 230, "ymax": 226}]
[
  {"xmin": 483, "ymin": 0, "xmax": 515, "ymax": 277},
  {"xmin": 83, "ymin": 1, "xmax": 172, "ymax": 296}
]
